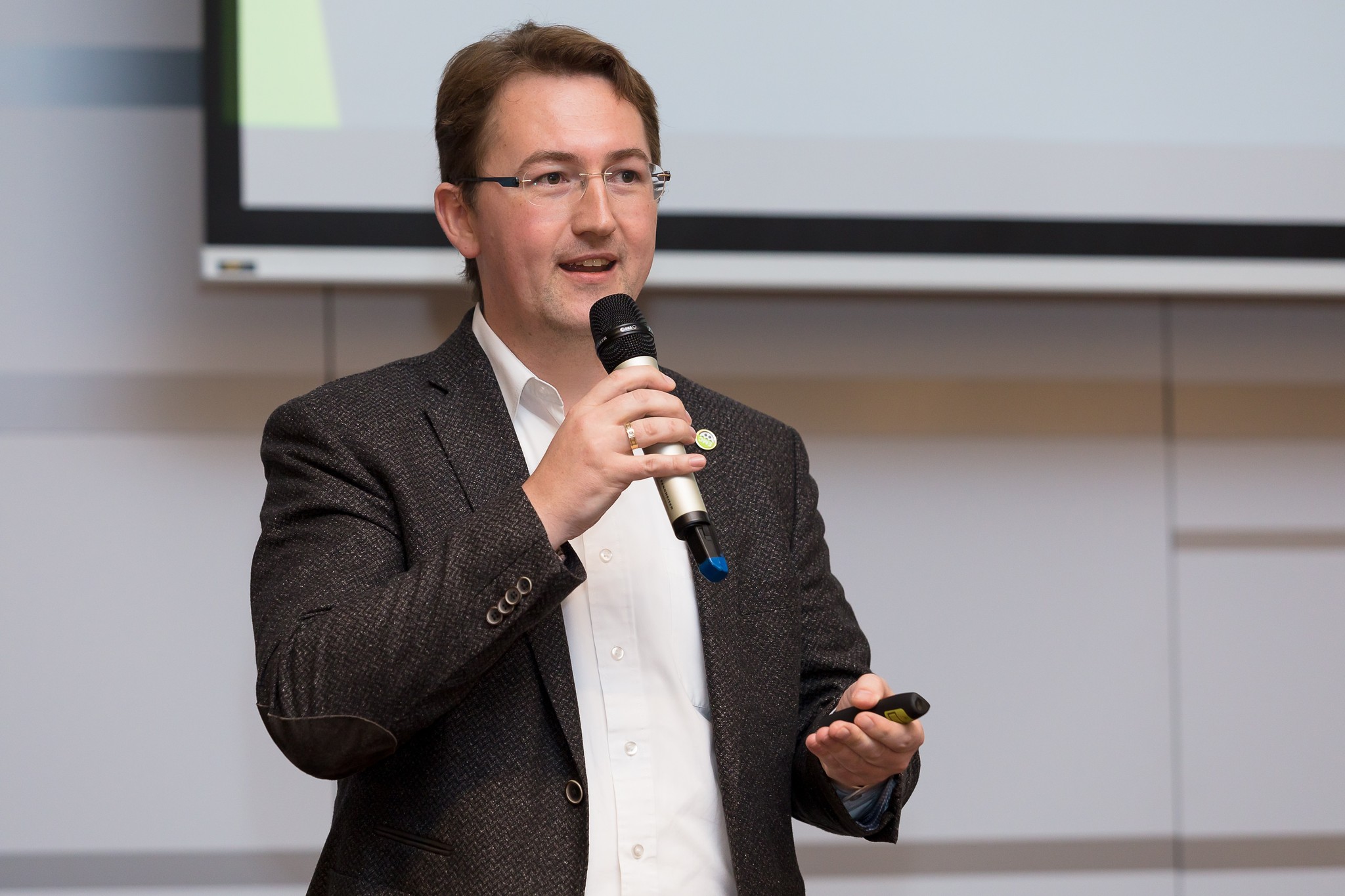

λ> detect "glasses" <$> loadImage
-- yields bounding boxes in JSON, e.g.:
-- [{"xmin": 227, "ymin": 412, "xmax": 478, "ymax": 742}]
[{"xmin": 453, "ymin": 161, "xmax": 670, "ymax": 208}]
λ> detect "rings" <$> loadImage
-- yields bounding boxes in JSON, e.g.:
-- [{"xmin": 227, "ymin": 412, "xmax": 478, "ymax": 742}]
[{"xmin": 625, "ymin": 424, "xmax": 641, "ymax": 448}]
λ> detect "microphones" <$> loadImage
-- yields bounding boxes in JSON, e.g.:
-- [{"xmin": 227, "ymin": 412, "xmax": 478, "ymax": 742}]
[{"xmin": 589, "ymin": 293, "xmax": 729, "ymax": 584}]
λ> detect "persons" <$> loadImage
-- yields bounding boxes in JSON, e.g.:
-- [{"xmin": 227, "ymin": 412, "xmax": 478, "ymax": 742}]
[{"xmin": 249, "ymin": 24, "xmax": 930, "ymax": 896}]
